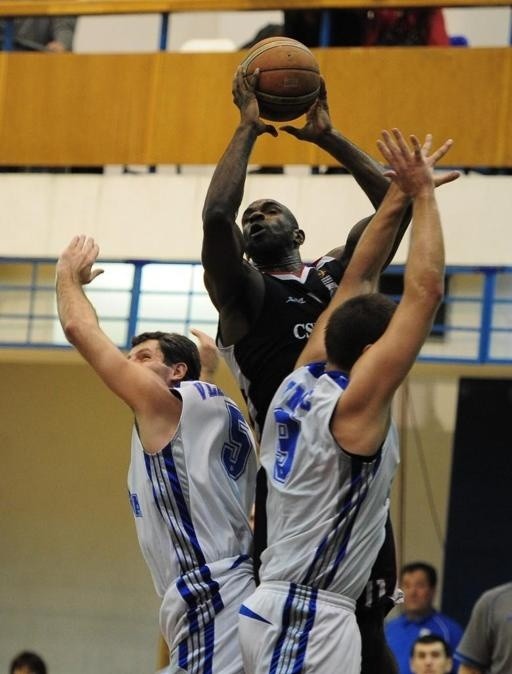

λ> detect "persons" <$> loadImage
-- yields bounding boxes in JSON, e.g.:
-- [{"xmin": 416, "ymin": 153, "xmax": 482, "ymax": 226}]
[
  {"xmin": 454, "ymin": 582, "xmax": 512, "ymax": 674},
  {"xmin": 202, "ymin": 66, "xmax": 413, "ymax": 673},
  {"xmin": 10, "ymin": 652, "xmax": 48, "ymax": 674},
  {"xmin": 53, "ymin": 234, "xmax": 256, "ymax": 674},
  {"xmin": 237, "ymin": 127, "xmax": 459, "ymax": 673},
  {"xmin": 382, "ymin": 563, "xmax": 463, "ymax": 672},
  {"xmin": 409, "ymin": 635, "xmax": 452, "ymax": 673}
]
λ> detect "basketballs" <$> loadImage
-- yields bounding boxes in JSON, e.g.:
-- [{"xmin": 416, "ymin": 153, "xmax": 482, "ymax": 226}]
[{"xmin": 239, "ymin": 34, "xmax": 323, "ymax": 123}]
